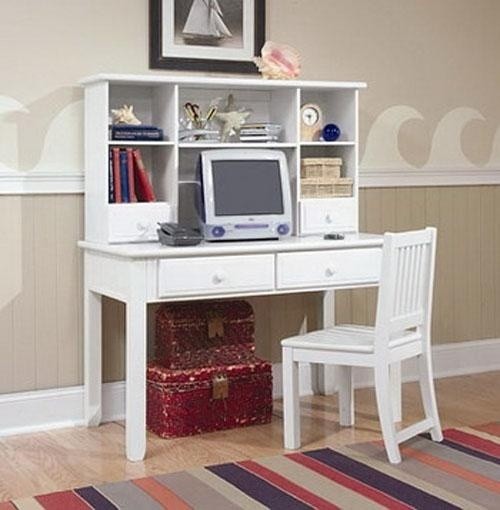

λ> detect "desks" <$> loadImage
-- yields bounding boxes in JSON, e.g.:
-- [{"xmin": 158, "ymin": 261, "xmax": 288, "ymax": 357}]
[{"xmin": 77, "ymin": 232, "xmax": 393, "ymax": 459}]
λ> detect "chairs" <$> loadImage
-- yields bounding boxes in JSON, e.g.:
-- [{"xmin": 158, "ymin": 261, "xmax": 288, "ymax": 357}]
[{"xmin": 279, "ymin": 227, "xmax": 445, "ymax": 467}]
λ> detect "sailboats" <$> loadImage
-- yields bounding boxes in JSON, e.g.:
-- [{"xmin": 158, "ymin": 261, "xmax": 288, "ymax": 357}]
[{"xmin": 182, "ymin": 0, "xmax": 233, "ymax": 46}]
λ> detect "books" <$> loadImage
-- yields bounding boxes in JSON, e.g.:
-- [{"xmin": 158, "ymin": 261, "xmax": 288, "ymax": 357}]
[{"xmin": 108, "ymin": 125, "xmax": 163, "ymax": 202}]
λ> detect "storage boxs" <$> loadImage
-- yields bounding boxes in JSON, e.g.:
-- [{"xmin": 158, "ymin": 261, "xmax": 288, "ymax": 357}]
[{"xmin": 144, "ymin": 299, "xmax": 273, "ymax": 439}]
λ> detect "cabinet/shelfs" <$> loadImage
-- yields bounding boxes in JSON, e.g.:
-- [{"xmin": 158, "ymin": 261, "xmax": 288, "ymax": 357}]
[{"xmin": 82, "ymin": 72, "xmax": 363, "ymax": 241}]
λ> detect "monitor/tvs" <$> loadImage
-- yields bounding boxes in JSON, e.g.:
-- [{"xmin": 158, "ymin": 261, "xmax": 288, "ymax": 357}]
[{"xmin": 194, "ymin": 147, "xmax": 294, "ymax": 242}]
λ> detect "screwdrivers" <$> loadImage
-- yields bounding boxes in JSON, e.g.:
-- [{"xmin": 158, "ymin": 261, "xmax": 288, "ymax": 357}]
[{"xmin": 185, "ymin": 102, "xmax": 217, "ymax": 141}]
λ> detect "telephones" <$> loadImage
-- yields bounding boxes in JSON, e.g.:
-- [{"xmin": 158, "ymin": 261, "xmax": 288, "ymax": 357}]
[{"xmin": 157, "ymin": 222, "xmax": 202, "ymax": 245}]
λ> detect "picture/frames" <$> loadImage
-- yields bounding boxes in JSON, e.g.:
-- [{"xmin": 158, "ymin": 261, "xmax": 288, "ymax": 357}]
[{"xmin": 147, "ymin": 0, "xmax": 268, "ymax": 75}]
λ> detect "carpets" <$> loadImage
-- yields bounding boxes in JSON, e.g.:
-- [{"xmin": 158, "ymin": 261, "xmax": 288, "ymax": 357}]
[{"xmin": 0, "ymin": 423, "xmax": 500, "ymax": 509}]
